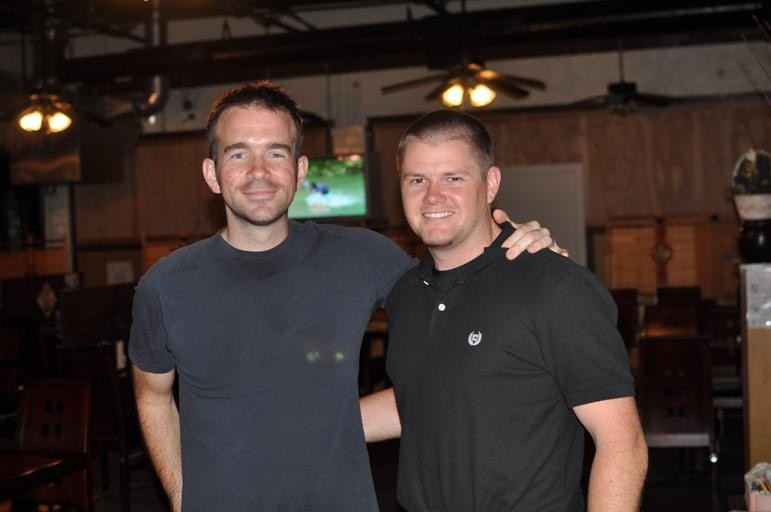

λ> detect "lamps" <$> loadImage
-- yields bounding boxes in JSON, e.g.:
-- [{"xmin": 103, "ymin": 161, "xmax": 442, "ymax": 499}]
[
  {"xmin": 14, "ymin": 81, "xmax": 72, "ymax": 134},
  {"xmin": 438, "ymin": 78, "xmax": 497, "ymax": 109},
  {"xmin": 605, "ymin": 99, "xmax": 639, "ymax": 115}
]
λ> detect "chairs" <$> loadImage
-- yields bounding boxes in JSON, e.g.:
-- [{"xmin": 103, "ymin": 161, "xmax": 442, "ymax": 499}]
[
  {"xmin": 2, "ymin": 279, "xmax": 164, "ymax": 509},
  {"xmin": 608, "ymin": 282, "xmax": 746, "ymax": 512}
]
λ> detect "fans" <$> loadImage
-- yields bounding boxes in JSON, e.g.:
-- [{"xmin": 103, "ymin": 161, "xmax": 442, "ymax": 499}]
[
  {"xmin": 381, "ymin": 0, "xmax": 547, "ymax": 102},
  {"xmin": 569, "ymin": 18, "xmax": 670, "ymax": 109}
]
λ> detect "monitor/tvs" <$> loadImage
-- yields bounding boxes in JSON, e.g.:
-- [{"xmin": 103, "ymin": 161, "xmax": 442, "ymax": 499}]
[{"xmin": 287, "ymin": 152, "xmax": 371, "ymax": 224}]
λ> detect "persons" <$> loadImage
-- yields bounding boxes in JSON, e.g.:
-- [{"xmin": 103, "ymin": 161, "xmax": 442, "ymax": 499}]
[
  {"xmin": 360, "ymin": 109, "xmax": 649, "ymax": 512},
  {"xmin": 130, "ymin": 84, "xmax": 419, "ymax": 511}
]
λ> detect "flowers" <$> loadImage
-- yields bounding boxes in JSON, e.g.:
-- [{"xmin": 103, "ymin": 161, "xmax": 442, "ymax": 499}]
[{"xmin": 727, "ymin": 143, "xmax": 770, "ymax": 196}]
